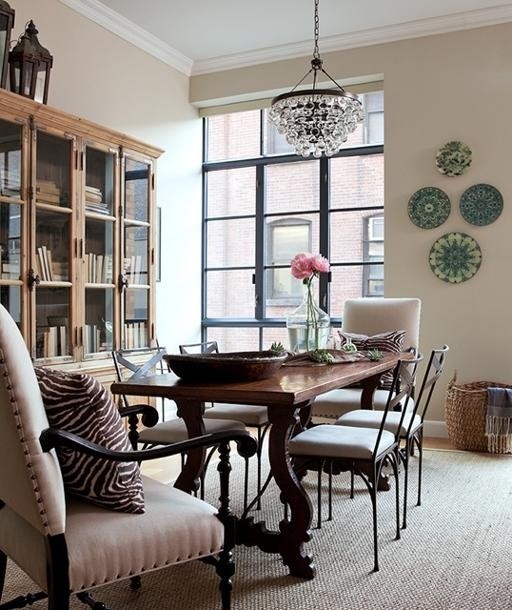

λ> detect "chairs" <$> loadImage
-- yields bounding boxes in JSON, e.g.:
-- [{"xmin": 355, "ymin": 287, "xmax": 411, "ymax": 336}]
[
  {"xmin": 283, "ymin": 351, "xmax": 424, "ymax": 574},
  {"xmin": 110, "ymin": 341, "xmax": 273, "ymax": 513},
  {"xmin": 308, "ymin": 296, "xmax": 422, "ymax": 475},
  {"xmin": 0, "ymin": 300, "xmax": 258, "ymax": 610},
  {"xmin": 333, "ymin": 344, "xmax": 449, "ymax": 530}
]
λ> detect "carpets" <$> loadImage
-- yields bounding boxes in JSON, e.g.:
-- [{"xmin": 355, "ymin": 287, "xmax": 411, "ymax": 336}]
[{"xmin": 1, "ymin": 422, "xmax": 511, "ymax": 610}]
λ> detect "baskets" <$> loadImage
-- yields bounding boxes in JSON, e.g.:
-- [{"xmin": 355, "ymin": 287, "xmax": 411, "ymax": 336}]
[
  {"xmin": 445, "ymin": 369, "xmax": 511, "ymax": 454},
  {"xmin": 162, "ymin": 350, "xmax": 288, "ymax": 383}
]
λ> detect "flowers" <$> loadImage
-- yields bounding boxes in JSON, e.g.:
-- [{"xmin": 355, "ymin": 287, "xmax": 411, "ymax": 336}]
[{"xmin": 289, "ymin": 250, "xmax": 332, "ymax": 349}]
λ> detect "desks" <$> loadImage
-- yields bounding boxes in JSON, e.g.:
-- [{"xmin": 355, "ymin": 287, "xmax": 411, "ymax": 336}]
[{"xmin": 111, "ymin": 344, "xmax": 417, "ymax": 581}]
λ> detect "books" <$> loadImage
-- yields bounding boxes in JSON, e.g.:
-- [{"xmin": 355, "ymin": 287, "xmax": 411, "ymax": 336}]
[{"xmin": 0, "ymin": 180, "xmax": 149, "ymax": 357}]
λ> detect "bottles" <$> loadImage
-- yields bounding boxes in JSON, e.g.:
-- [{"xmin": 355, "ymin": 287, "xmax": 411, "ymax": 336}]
[{"xmin": 342, "ymin": 337, "xmax": 357, "ymax": 352}]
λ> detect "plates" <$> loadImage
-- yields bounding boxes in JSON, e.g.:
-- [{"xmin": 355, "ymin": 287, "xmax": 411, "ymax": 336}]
[
  {"xmin": 459, "ymin": 183, "xmax": 503, "ymax": 225},
  {"xmin": 407, "ymin": 187, "xmax": 451, "ymax": 230},
  {"xmin": 435, "ymin": 140, "xmax": 472, "ymax": 177},
  {"xmin": 429, "ymin": 232, "xmax": 482, "ymax": 283}
]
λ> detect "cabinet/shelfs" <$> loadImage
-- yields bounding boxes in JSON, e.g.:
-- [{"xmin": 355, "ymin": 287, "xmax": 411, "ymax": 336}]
[{"xmin": 0, "ymin": 85, "xmax": 157, "ymax": 435}]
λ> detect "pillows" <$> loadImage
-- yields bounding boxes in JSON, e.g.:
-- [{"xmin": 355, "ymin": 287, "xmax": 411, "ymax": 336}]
[
  {"xmin": 35, "ymin": 366, "xmax": 146, "ymax": 516},
  {"xmin": 336, "ymin": 328, "xmax": 405, "ymax": 393}
]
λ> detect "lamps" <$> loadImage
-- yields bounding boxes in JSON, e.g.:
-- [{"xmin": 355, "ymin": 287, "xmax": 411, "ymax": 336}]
[
  {"xmin": 7, "ymin": 19, "xmax": 53, "ymax": 105},
  {"xmin": 265, "ymin": 0, "xmax": 367, "ymax": 160}
]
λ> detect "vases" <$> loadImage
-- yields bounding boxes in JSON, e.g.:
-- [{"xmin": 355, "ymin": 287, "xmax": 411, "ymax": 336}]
[{"xmin": 286, "ymin": 284, "xmax": 331, "ymax": 355}]
